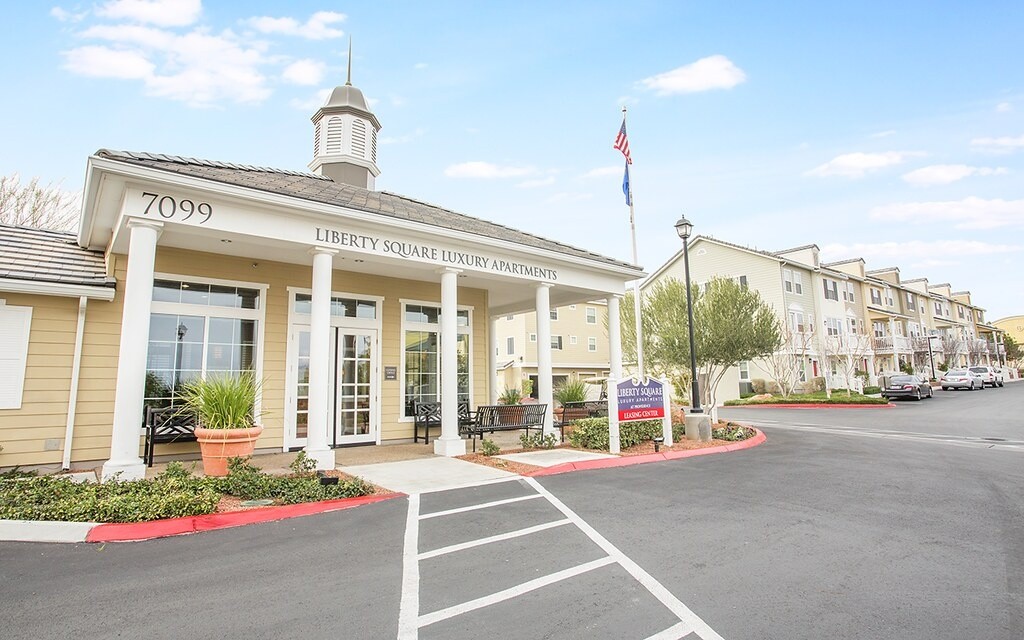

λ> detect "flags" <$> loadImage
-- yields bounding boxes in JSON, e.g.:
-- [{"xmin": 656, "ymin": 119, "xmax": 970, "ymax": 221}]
[{"xmin": 613, "ymin": 119, "xmax": 633, "ymax": 207}]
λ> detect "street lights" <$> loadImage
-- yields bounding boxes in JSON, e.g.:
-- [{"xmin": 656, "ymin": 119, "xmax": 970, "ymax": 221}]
[
  {"xmin": 996, "ymin": 343, "xmax": 1004, "ymax": 368},
  {"xmin": 674, "ymin": 213, "xmax": 712, "ymax": 443},
  {"xmin": 928, "ymin": 336, "xmax": 937, "ymax": 381},
  {"xmin": 173, "ymin": 321, "xmax": 188, "ymax": 386}
]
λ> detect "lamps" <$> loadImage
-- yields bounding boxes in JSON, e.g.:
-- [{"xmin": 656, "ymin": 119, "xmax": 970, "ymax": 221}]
[
  {"xmin": 653, "ymin": 437, "xmax": 665, "ymax": 443},
  {"xmin": 321, "ymin": 477, "xmax": 339, "ymax": 485}
]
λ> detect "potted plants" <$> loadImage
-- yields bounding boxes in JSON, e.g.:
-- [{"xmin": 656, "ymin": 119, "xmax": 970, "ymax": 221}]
[
  {"xmin": 158, "ymin": 360, "xmax": 275, "ymax": 479},
  {"xmin": 495, "ymin": 383, "xmax": 524, "ymax": 426},
  {"xmin": 552, "ymin": 374, "xmax": 592, "ymax": 432}
]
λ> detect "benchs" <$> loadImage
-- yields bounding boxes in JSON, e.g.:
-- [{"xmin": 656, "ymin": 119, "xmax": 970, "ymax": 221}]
[
  {"xmin": 144, "ymin": 405, "xmax": 198, "ymax": 468},
  {"xmin": 457, "ymin": 404, "xmax": 548, "ymax": 453},
  {"xmin": 553, "ymin": 399, "xmax": 609, "ymax": 443},
  {"xmin": 414, "ymin": 401, "xmax": 483, "ymax": 446}
]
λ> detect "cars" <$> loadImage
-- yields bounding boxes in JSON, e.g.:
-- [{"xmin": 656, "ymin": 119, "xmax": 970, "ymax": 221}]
[
  {"xmin": 969, "ymin": 366, "xmax": 1004, "ymax": 387},
  {"xmin": 881, "ymin": 374, "xmax": 934, "ymax": 400},
  {"xmin": 941, "ymin": 370, "xmax": 986, "ymax": 391}
]
